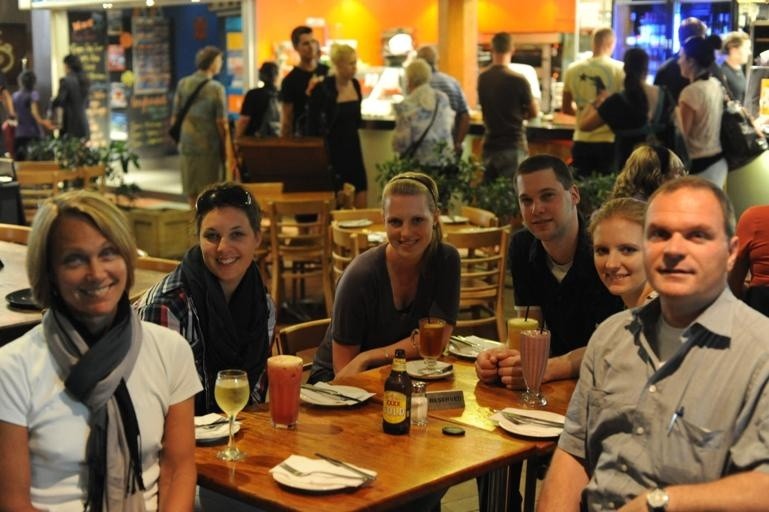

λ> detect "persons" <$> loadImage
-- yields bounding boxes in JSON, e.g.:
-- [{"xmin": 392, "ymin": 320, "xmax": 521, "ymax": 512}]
[
  {"xmin": 277, "ymin": 26, "xmax": 334, "ymax": 138},
  {"xmin": 0, "ymin": 188, "xmax": 204, "ymax": 512},
  {"xmin": 562, "ymin": 17, "xmax": 752, "ymax": 193},
  {"xmin": 611, "ymin": 143, "xmax": 689, "ymax": 203},
  {"xmin": 729, "ymin": 204, "xmax": 769, "ymax": 317},
  {"xmin": 589, "ymin": 197, "xmax": 654, "ymax": 309},
  {"xmin": 170, "ymin": 45, "xmax": 237, "ymax": 208},
  {"xmin": 11, "ymin": 69, "xmax": 59, "ymax": 161},
  {"xmin": 50, "ymin": 53, "xmax": 91, "ymax": 158},
  {"xmin": 391, "ymin": 45, "xmax": 471, "ymax": 212},
  {"xmin": 137, "ymin": 182, "xmax": 277, "ymax": 416},
  {"xmin": 233, "ymin": 62, "xmax": 282, "ymax": 182},
  {"xmin": 473, "ymin": 154, "xmax": 625, "ymax": 392},
  {"xmin": 308, "ymin": 42, "xmax": 368, "ymax": 210},
  {"xmin": 535, "ymin": 176, "xmax": 768, "ymax": 511},
  {"xmin": 1, "ymin": 72, "xmax": 17, "ymax": 158},
  {"xmin": 307, "ymin": 172, "xmax": 461, "ymax": 387},
  {"xmin": 477, "ymin": 32, "xmax": 542, "ymax": 187}
]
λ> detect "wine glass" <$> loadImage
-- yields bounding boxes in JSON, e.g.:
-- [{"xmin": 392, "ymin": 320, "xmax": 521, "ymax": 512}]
[
  {"xmin": 215, "ymin": 369, "xmax": 251, "ymax": 464},
  {"xmin": 416, "ymin": 320, "xmax": 448, "ymax": 375}
]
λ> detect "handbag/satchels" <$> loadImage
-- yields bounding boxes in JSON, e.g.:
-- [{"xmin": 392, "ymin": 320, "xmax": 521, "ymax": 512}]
[
  {"xmin": 168, "ymin": 115, "xmax": 182, "ymax": 142},
  {"xmin": 720, "ymin": 101, "xmax": 768, "ymax": 171}
]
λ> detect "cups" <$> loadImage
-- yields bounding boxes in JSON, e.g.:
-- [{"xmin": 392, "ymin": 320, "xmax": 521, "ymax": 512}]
[
  {"xmin": 266, "ymin": 355, "xmax": 303, "ymax": 427},
  {"xmin": 520, "ymin": 327, "xmax": 552, "ymax": 406},
  {"xmin": 508, "ymin": 318, "xmax": 538, "ymax": 353}
]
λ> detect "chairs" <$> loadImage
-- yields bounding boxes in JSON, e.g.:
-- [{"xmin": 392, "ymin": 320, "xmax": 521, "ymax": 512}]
[
  {"xmin": 12, "ymin": 162, "xmax": 105, "ymax": 232},
  {"xmin": 233, "ymin": 183, "xmax": 513, "ymax": 372}
]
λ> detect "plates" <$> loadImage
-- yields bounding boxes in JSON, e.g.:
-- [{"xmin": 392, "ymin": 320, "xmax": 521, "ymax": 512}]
[
  {"xmin": 274, "ymin": 466, "xmax": 362, "ymax": 494},
  {"xmin": 406, "ymin": 360, "xmax": 454, "ymax": 377},
  {"xmin": 500, "ymin": 408, "xmax": 567, "ymax": 440},
  {"xmin": 438, "ymin": 214, "xmax": 469, "ymax": 225},
  {"xmin": 305, "ymin": 384, "xmax": 371, "ymax": 409},
  {"xmin": 195, "ymin": 419, "xmax": 241, "ymax": 443},
  {"xmin": 446, "ymin": 336, "xmax": 508, "ymax": 358},
  {"xmin": 340, "ymin": 219, "xmax": 375, "ymax": 230},
  {"xmin": 5, "ymin": 287, "xmax": 41, "ymax": 310}
]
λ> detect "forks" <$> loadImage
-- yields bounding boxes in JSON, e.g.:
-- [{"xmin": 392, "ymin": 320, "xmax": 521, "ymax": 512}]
[{"xmin": 279, "ymin": 463, "xmax": 361, "ymax": 481}]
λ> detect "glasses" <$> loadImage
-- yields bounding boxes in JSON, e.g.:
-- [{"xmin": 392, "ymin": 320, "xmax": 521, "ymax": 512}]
[{"xmin": 196, "ymin": 187, "xmax": 251, "ymax": 210}]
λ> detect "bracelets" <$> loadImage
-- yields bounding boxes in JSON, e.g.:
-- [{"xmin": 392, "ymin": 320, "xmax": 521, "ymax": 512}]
[{"xmin": 381, "ymin": 347, "xmax": 389, "ymax": 365}]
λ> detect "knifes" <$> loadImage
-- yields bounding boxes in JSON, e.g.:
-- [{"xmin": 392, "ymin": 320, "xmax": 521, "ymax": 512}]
[
  {"xmin": 302, "ymin": 383, "xmax": 363, "ymax": 404},
  {"xmin": 315, "ymin": 452, "xmax": 374, "ymax": 480},
  {"xmin": 506, "ymin": 412, "xmax": 564, "ymax": 428}
]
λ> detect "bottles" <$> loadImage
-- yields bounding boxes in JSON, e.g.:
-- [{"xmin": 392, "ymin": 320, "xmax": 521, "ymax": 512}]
[
  {"xmin": 410, "ymin": 378, "xmax": 431, "ymax": 424},
  {"xmin": 382, "ymin": 348, "xmax": 412, "ymax": 435}
]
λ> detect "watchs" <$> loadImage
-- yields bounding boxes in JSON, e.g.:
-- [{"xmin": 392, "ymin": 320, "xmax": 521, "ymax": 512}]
[{"xmin": 646, "ymin": 487, "xmax": 669, "ymax": 512}]
